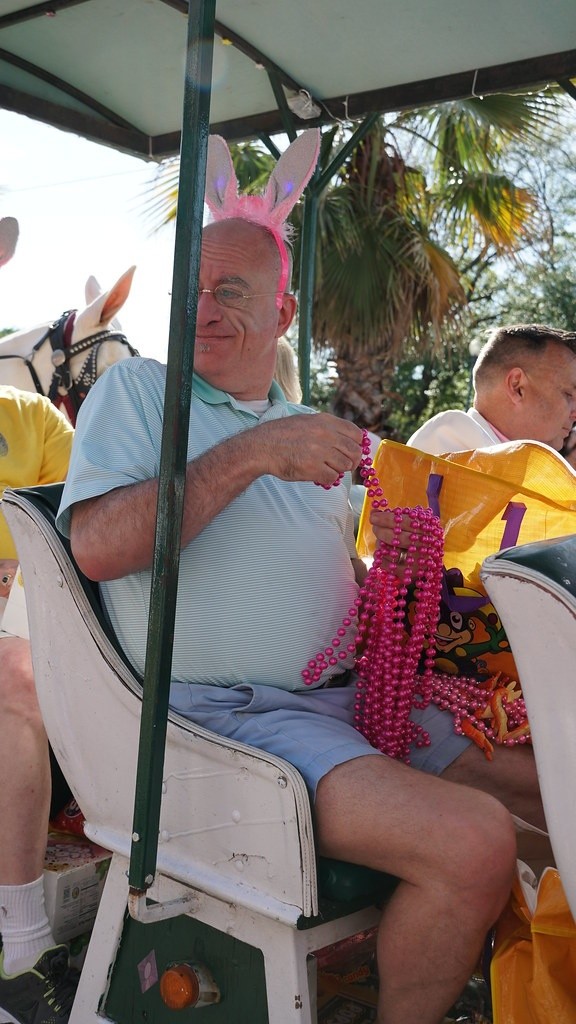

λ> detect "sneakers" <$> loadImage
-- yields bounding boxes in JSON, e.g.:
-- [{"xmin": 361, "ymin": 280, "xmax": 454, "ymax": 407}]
[{"xmin": 0, "ymin": 944, "xmax": 78, "ymax": 1024}]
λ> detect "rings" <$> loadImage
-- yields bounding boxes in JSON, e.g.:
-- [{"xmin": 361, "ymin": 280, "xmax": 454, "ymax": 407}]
[{"xmin": 396, "ymin": 548, "xmax": 408, "ymax": 566}]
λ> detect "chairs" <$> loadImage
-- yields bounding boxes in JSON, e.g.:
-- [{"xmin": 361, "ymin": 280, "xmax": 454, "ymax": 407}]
[
  {"xmin": 0, "ymin": 483, "xmax": 403, "ymax": 1024},
  {"xmin": 479, "ymin": 536, "xmax": 575, "ymax": 922}
]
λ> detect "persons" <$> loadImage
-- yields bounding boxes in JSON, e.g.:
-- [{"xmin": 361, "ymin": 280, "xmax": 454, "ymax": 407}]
[
  {"xmin": 0, "ymin": 384, "xmax": 80, "ymax": 1022},
  {"xmin": 403, "ymin": 326, "xmax": 576, "ymax": 470},
  {"xmin": 55, "ymin": 216, "xmax": 548, "ymax": 1021}
]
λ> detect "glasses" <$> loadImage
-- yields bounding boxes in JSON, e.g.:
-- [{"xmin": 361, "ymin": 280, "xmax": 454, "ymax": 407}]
[{"xmin": 198, "ymin": 284, "xmax": 287, "ymax": 306}]
